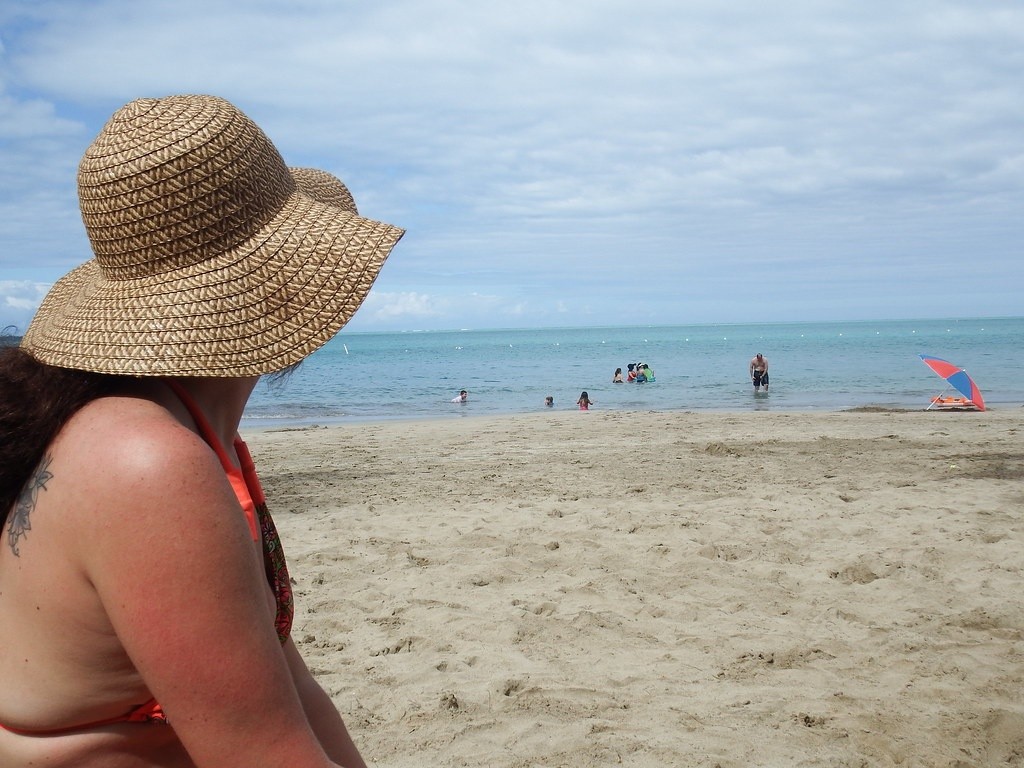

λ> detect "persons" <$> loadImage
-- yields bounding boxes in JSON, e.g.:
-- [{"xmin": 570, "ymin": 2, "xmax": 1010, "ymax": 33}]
[
  {"xmin": 0, "ymin": 98, "xmax": 408, "ymax": 768},
  {"xmin": 613, "ymin": 368, "xmax": 624, "ymax": 383},
  {"xmin": 577, "ymin": 391, "xmax": 593, "ymax": 410},
  {"xmin": 637, "ymin": 363, "xmax": 656, "ymax": 382},
  {"xmin": 545, "ymin": 396, "xmax": 553, "ymax": 407},
  {"xmin": 750, "ymin": 353, "xmax": 769, "ymax": 391},
  {"xmin": 627, "ymin": 364, "xmax": 636, "ymax": 381},
  {"xmin": 451, "ymin": 390, "xmax": 467, "ymax": 402}
]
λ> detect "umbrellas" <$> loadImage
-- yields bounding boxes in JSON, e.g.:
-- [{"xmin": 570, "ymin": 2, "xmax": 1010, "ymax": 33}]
[{"xmin": 919, "ymin": 353, "xmax": 985, "ymax": 412}]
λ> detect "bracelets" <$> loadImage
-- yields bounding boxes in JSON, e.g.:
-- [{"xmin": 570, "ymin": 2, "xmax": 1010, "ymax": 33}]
[{"xmin": 762, "ymin": 376, "xmax": 763, "ymax": 378}]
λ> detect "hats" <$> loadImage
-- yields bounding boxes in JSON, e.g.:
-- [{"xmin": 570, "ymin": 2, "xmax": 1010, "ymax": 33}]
[
  {"xmin": 628, "ymin": 363, "xmax": 636, "ymax": 371},
  {"xmin": 17, "ymin": 92, "xmax": 404, "ymax": 377},
  {"xmin": 638, "ymin": 363, "xmax": 644, "ymax": 367}
]
